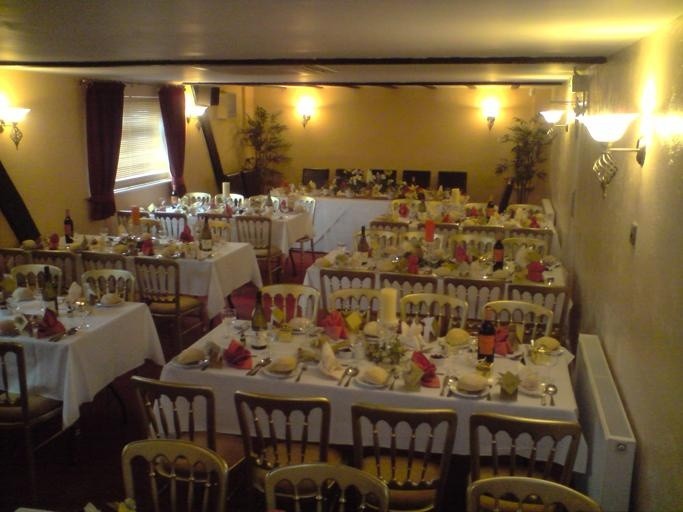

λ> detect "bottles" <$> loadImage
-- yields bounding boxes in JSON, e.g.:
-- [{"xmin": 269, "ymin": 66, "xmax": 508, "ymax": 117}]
[
  {"xmin": 64, "ymin": 209, "xmax": 74, "ymax": 244},
  {"xmin": 357, "ymin": 226, "xmax": 369, "ymax": 265},
  {"xmin": 42, "ymin": 266, "xmax": 58, "ymax": 316},
  {"xmin": 251, "ymin": 291, "xmax": 268, "ymax": 350},
  {"xmin": 170, "ymin": 185, "xmax": 179, "ymax": 209},
  {"xmin": 265, "ymin": 191, "xmax": 273, "ymax": 216},
  {"xmin": 417, "ymin": 198, "xmax": 427, "ymax": 221},
  {"xmin": 323, "ymin": 177, "xmax": 330, "ymax": 197},
  {"xmin": 477, "ymin": 306, "xmax": 495, "ymax": 365},
  {"xmin": 444, "ymin": 174, "xmax": 452, "ymax": 196},
  {"xmin": 486, "ymin": 194, "xmax": 497, "ymax": 223},
  {"xmin": 200, "ymin": 216, "xmax": 211, "ymax": 252},
  {"xmin": 493, "ymin": 232, "xmax": 504, "ymax": 270},
  {"xmin": 0, "ymin": 287, "xmax": 8, "ymax": 309}
]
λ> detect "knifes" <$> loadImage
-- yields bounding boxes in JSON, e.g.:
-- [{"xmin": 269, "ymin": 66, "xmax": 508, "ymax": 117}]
[{"xmin": 439, "ymin": 374, "xmax": 449, "ymax": 397}]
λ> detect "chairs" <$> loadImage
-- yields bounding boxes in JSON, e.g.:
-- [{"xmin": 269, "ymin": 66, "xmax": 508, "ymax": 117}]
[
  {"xmin": 506, "ymin": 203, "xmax": 542, "ymax": 213},
  {"xmin": 379, "ymin": 273, "xmax": 437, "ymax": 326},
  {"xmin": 132, "ymin": 374, "xmax": 255, "ymax": 512},
  {"xmin": 10, "ymin": 264, "xmax": 62, "ymax": 298},
  {"xmin": 183, "ymin": 191, "xmax": 212, "ymax": 204},
  {"xmin": 264, "ymin": 464, "xmax": 388, "ymax": 511},
  {"xmin": 427, "ymin": 200, "xmax": 462, "ymax": 214},
  {"xmin": 508, "ymin": 284, "xmax": 570, "ymax": 349},
  {"xmin": 369, "ymin": 221, "xmax": 410, "ymax": 235},
  {"xmin": 391, "ymin": 200, "xmax": 423, "ymax": 215},
  {"xmin": 334, "ymin": 168, "xmax": 350, "ymax": 181},
  {"xmin": 277, "ymin": 195, "xmax": 316, "ymax": 268},
  {"xmin": 318, "ymin": 268, "xmax": 376, "ymax": 316},
  {"xmin": 397, "ymin": 232, "xmax": 445, "ymax": 258},
  {"xmin": 32, "ymin": 248, "xmax": 80, "ymax": 295},
  {"xmin": 402, "ymin": 170, "xmax": 432, "ymax": 192},
  {"xmin": 80, "ymin": 269, "xmax": 135, "ymax": 304},
  {"xmin": 399, "ymin": 293, "xmax": 470, "ymax": 339},
  {"xmin": 193, "ymin": 222, "xmax": 235, "ymax": 244},
  {"xmin": 301, "ymin": 168, "xmax": 328, "ymax": 190},
  {"xmin": 462, "ymin": 225, "xmax": 506, "ymax": 242},
  {"xmin": 468, "ymin": 414, "xmax": 581, "ymax": 512},
  {"xmin": 116, "ymin": 211, "xmax": 151, "ymax": 235},
  {"xmin": 154, "ymin": 212, "xmax": 188, "ymax": 239},
  {"xmin": 80, "ymin": 251, "xmax": 143, "ymax": 302},
  {"xmin": 351, "ymin": 405, "xmax": 459, "ymax": 512},
  {"xmin": 369, "ymin": 168, "xmax": 397, "ymax": 184},
  {"xmin": 197, "ymin": 211, "xmax": 232, "ymax": 244},
  {"xmin": 129, "ymin": 218, "xmax": 164, "ymax": 237},
  {"xmin": 132, "ymin": 255, "xmax": 206, "ymax": 352},
  {"xmin": 464, "ymin": 202, "xmax": 499, "ymax": 214},
  {"xmin": 0, "ymin": 341, "xmax": 64, "ymax": 482},
  {"xmin": 0, "ymin": 246, "xmax": 36, "ymax": 289},
  {"xmin": 247, "ymin": 195, "xmax": 281, "ymax": 211},
  {"xmin": 509, "ymin": 227, "xmax": 552, "ymax": 254},
  {"xmin": 122, "ymin": 440, "xmax": 227, "ymax": 512},
  {"xmin": 416, "ymin": 223, "xmax": 458, "ymax": 236},
  {"xmin": 437, "ymin": 170, "xmax": 467, "ymax": 195},
  {"xmin": 327, "ymin": 288, "xmax": 382, "ymax": 329},
  {"xmin": 502, "ymin": 237, "xmax": 548, "ymax": 261},
  {"xmin": 481, "ymin": 300, "xmax": 554, "ymax": 347},
  {"xmin": 235, "ymin": 393, "xmax": 347, "ymax": 512},
  {"xmin": 235, "ymin": 215, "xmax": 290, "ymax": 286},
  {"xmin": 447, "ymin": 235, "xmax": 498, "ymax": 259},
  {"xmin": 352, "ymin": 231, "xmax": 397, "ymax": 253},
  {"xmin": 214, "ymin": 192, "xmax": 244, "ymax": 207},
  {"xmin": 253, "ymin": 284, "xmax": 321, "ymax": 326},
  {"xmin": 467, "ymin": 475, "xmax": 601, "ymax": 512},
  {"xmin": 443, "ymin": 276, "xmax": 505, "ymax": 335}
]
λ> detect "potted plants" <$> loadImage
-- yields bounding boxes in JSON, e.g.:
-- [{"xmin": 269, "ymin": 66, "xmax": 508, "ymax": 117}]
[{"xmin": 236, "ymin": 106, "xmax": 292, "ymax": 200}]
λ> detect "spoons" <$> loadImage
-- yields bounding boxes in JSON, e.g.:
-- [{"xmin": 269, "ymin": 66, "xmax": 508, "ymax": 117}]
[
  {"xmin": 184, "ymin": 359, "xmax": 209, "ymax": 368},
  {"xmin": 51, "ymin": 327, "xmax": 78, "ymax": 342},
  {"xmin": 446, "ymin": 375, "xmax": 458, "ymax": 397},
  {"xmin": 247, "ymin": 358, "xmax": 269, "ymax": 374},
  {"xmin": 343, "ymin": 366, "xmax": 360, "ymax": 387},
  {"xmin": 546, "ymin": 383, "xmax": 558, "ymax": 406}
]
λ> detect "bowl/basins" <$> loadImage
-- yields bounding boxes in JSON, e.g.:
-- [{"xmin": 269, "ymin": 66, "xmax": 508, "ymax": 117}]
[
  {"xmin": 430, "ymin": 352, "xmax": 445, "ymax": 367},
  {"xmin": 338, "ymin": 346, "xmax": 352, "ymax": 359}
]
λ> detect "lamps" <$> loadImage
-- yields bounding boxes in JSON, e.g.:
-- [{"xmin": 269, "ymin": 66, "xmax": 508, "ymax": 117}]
[
  {"xmin": 540, "ymin": 109, "xmax": 568, "ymax": 137},
  {"xmin": 483, "ymin": 100, "xmax": 499, "ymax": 128},
  {"xmin": 185, "ymin": 92, "xmax": 207, "ymax": 131},
  {"xmin": 576, "ymin": 110, "xmax": 646, "ymax": 190},
  {"xmin": 297, "ymin": 95, "xmax": 315, "ymax": 129},
  {"xmin": 2, "ymin": 106, "xmax": 31, "ymax": 151}
]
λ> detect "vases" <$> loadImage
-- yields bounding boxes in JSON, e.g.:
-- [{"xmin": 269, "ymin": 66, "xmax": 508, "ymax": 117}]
[
  {"xmin": 343, "ymin": 186, "xmax": 357, "ymax": 198},
  {"xmin": 385, "ymin": 187, "xmax": 397, "ymax": 199}
]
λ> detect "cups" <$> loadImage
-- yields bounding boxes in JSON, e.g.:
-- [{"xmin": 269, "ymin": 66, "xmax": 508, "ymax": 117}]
[
  {"xmin": 540, "ymin": 350, "xmax": 558, "ymax": 381},
  {"xmin": 222, "ymin": 310, "xmax": 234, "ymax": 340},
  {"xmin": 468, "ymin": 331, "xmax": 478, "ymax": 364},
  {"xmin": 267, "ymin": 342, "xmax": 298, "ymax": 371},
  {"xmin": 158, "ymin": 197, "xmax": 167, "ymax": 208},
  {"xmin": 73, "ymin": 296, "xmax": 90, "ymax": 330}
]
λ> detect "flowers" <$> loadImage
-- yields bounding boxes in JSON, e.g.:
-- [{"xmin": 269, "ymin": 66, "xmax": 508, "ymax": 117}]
[
  {"xmin": 374, "ymin": 170, "xmax": 408, "ymax": 195},
  {"xmin": 330, "ymin": 167, "xmax": 376, "ymax": 196}
]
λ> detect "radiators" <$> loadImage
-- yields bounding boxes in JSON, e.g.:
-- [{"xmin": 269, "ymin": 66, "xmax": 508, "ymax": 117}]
[
  {"xmin": 574, "ymin": 334, "xmax": 636, "ymax": 512},
  {"xmin": 540, "ymin": 197, "xmax": 555, "ymax": 228}
]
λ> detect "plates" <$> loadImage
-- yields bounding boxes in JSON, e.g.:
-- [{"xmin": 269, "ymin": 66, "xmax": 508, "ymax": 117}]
[
  {"xmin": 16, "ymin": 301, "xmax": 44, "ymax": 315},
  {"xmin": 449, "ymin": 387, "xmax": 492, "ymax": 400},
  {"xmin": 171, "ymin": 356, "xmax": 211, "ymax": 369},
  {"xmin": 261, "ymin": 366, "xmax": 301, "ymax": 379},
  {"xmin": 355, "ymin": 374, "xmax": 395, "ymax": 389},
  {"xmin": 96, "ymin": 301, "xmax": 124, "ymax": 307}
]
